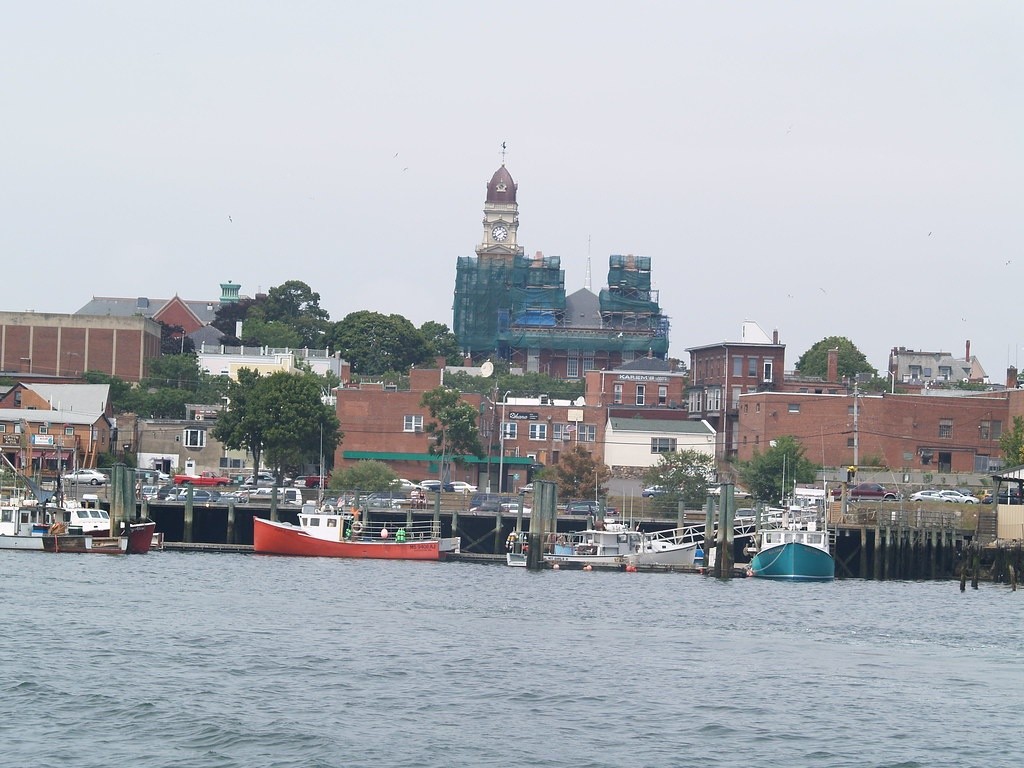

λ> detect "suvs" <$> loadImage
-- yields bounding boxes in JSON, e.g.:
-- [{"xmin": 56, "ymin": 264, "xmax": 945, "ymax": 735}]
[{"xmin": 566, "ymin": 500, "xmax": 620, "ymax": 516}]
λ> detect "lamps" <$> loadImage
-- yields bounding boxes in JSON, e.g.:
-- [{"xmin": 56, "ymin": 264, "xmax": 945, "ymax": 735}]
[{"xmin": 978, "ymin": 425, "xmax": 981, "ymax": 430}]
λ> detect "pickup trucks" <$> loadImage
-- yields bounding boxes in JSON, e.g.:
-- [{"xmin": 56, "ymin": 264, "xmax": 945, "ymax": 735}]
[
  {"xmin": 174, "ymin": 470, "xmax": 233, "ymax": 489},
  {"xmin": 830, "ymin": 482, "xmax": 901, "ymax": 502}
]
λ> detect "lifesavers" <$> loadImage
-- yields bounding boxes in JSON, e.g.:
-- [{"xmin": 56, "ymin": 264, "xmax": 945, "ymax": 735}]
[{"xmin": 350, "ymin": 522, "xmax": 363, "ymax": 534}]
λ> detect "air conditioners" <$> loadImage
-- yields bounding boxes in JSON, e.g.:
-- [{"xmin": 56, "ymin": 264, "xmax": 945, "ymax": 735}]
[
  {"xmin": 194, "ymin": 413, "xmax": 204, "ymax": 421},
  {"xmin": 562, "ymin": 432, "xmax": 570, "ymax": 440},
  {"xmin": 414, "ymin": 425, "xmax": 422, "ymax": 431}
]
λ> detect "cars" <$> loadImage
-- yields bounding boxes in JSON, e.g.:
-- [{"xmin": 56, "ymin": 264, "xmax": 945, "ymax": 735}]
[
  {"xmin": 239, "ymin": 472, "xmax": 330, "ymax": 491},
  {"xmin": 570, "ymin": 505, "xmax": 599, "ymax": 515},
  {"xmin": 157, "ymin": 469, "xmax": 170, "ymax": 480},
  {"xmin": 416, "ymin": 479, "xmax": 456, "ymax": 493},
  {"xmin": 1005, "ymin": 487, "xmax": 1024, "ymax": 502},
  {"xmin": 641, "ymin": 483, "xmax": 753, "ymax": 502},
  {"xmin": 940, "ymin": 489, "xmax": 979, "ymax": 505},
  {"xmin": 973, "ymin": 489, "xmax": 994, "ymax": 501},
  {"xmin": 448, "ymin": 481, "xmax": 480, "ymax": 494},
  {"xmin": 61, "ymin": 467, "xmax": 111, "ymax": 487},
  {"xmin": 141, "ymin": 485, "xmax": 220, "ymax": 503},
  {"xmin": 518, "ymin": 483, "xmax": 533, "ymax": 494},
  {"xmin": 388, "ymin": 477, "xmax": 433, "ymax": 495},
  {"xmin": 981, "ymin": 493, "xmax": 1021, "ymax": 505},
  {"xmin": 908, "ymin": 490, "xmax": 959, "ymax": 504},
  {"xmin": 470, "ymin": 490, "xmax": 533, "ymax": 512}
]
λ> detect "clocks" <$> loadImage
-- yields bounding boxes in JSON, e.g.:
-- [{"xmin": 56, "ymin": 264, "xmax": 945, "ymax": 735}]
[{"xmin": 492, "ymin": 226, "xmax": 507, "ymax": 241}]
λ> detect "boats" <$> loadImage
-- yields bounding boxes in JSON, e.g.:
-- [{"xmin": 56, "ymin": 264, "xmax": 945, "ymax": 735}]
[
  {"xmin": 505, "ymin": 498, "xmax": 698, "ymax": 571},
  {"xmin": 38, "ymin": 434, "xmax": 157, "ymax": 555},
  {"xmin": 745, "ymin": 451, "xmax": 838, "ymax": 583},
  {"xmin": 0, "ymin": 485, "xmax": 112, "ymax": 551},
  {"xmin": 251, "ymin": 422, "xmax": 442, "ymax": 561}
]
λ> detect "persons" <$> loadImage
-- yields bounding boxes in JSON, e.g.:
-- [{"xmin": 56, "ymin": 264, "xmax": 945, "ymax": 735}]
[
  {"xmin": 417, "ymin": 489, "xmax": 427, "ymax": 509},
  {"xmin": 410, "ymin": 488, "xmax": 419, "ymax": 508}
]
